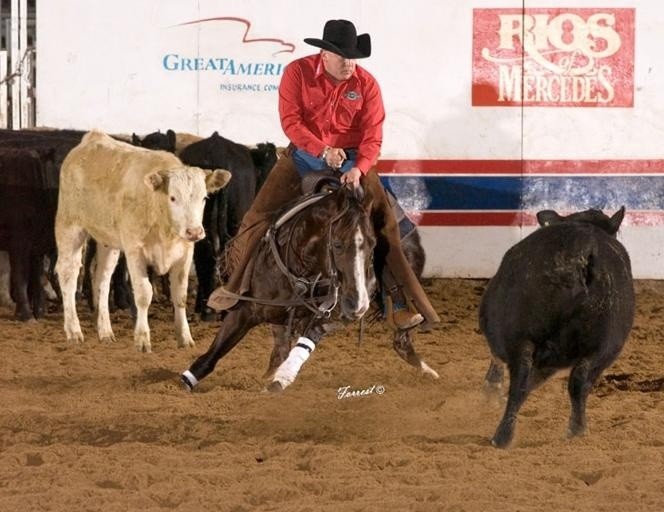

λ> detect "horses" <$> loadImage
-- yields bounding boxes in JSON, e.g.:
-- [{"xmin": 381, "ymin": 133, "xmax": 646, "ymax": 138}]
[{"xmin": 180, "ymin": 174, "xmax": 441, "ymax": 393}]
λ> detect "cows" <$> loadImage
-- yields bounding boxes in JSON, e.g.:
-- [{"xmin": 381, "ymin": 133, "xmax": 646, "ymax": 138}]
[
  {"xmin": 477, "ymin": 205, "xmax": 635, "ymax": 449},
  {"xmin": 0, "ymin": 125, "xmax": 285, "ymax": 354}
]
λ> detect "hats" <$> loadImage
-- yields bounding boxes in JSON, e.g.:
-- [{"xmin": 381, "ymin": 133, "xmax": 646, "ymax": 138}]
[{"xmin": 303, "ymin": 19, "xmax": 370, "ymax": 58}]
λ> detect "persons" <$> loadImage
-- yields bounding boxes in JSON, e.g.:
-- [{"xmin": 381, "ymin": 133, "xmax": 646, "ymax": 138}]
[{"xmin": 213, "ymin": 18, "xmax": 425, "ymax": 330}]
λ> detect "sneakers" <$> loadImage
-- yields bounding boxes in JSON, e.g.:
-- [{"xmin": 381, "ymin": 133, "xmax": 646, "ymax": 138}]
[{"xmin": 392, "ymin": 309, "xmax": 425, "ymax": 331}]
